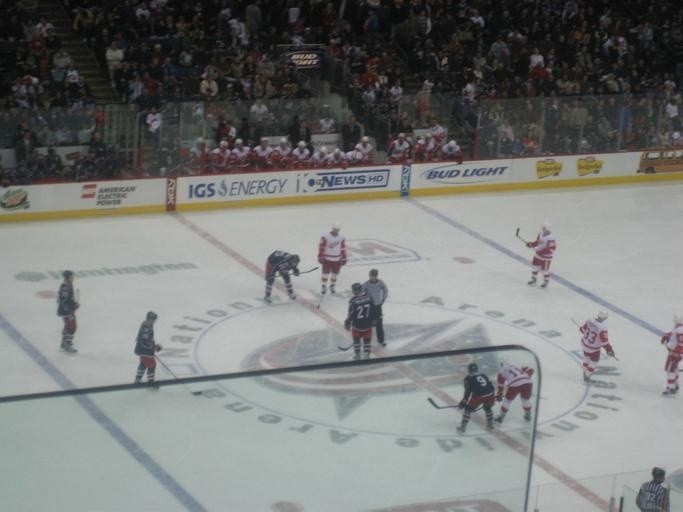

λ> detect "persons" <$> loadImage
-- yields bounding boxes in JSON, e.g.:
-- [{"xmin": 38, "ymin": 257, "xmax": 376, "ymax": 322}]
[
  {"xmin": 343, "ymin": 284, "xmax": 373, "ymax": 357},
  {"xmin": 527, "ymin": 222, "xmax": 556, "ymax": 284},
  {"xmin": 316, "ymin": 224, "xmax": 348, "ymax": 291},
  {"xmin": 457, "ymin": 362, "xmax": 499, "ymax": 432},
  {"xmin": 132, "ymin": 312, "xmax": 162, "ymax": 387},
  {"xmin": 635, "ymin": 463, "xmax": 671, "ymax": 509},
  {"xmin": 55, "ymin": 268, "xmax": 81, "ymax": 350},
  {"xmin": 259, "ymin": 251, "xmax": 302, "ymax": 301},
  {"xmin": 495, "ymin": 357, "xmax": 534, "ymax": 422},
  {"xmin": 1, "ymin": 0, "xmax": 683, "ymax": 180},
  {"xmin": 362, "ymin": 268, "xmax": 389, "ymax": 346},
  {"xmin": 659, "ymin": 312, "xmax": 680, "ymax": 395},
  {"xmin": 577, "ymin": 308, "xmax": 615, "ymax": 381}
]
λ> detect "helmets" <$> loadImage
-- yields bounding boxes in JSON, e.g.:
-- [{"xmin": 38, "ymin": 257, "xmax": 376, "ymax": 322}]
[
  {"xmin": 291, "ymin": 223, "xmax": 378, "ymax": 295},
  {"xmin": 146, "ymin": 311, "xmax": 157, "ymax": 324},
  {"xmin": 62, "ymin": 268, "xmax": 74, "ymax": 282},
  {"xmin": 651, "ymin": 468, "xmax": 666, "ymax": 484},
  {"xmin": 467, "ymin": 222, "xmax": 683, "ymax": 373},
  {"xmin": 220, "ymin": 133, "xmax": 455, "ymax": 155}
]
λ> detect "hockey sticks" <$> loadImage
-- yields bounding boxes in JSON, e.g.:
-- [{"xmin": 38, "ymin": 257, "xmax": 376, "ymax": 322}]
[
  {"xmin": 338, "ymin": 343, "xmax": 353, "ymax": 351},
  {"xmin": 570, "ymin": 316, "xmax": 620, "ymax": 361},
  {"xmin": 274, "ymin": 267, "xmax": 319, "ymax": 277},
  {"xmin": 428, "ymin": 397, "xmax": 460, "ymax": 409},
  {"xmin": 516, "ymin": 228, "xmax": 529, "ymax": 244},
  {"xmin": 153, "ymin": 353, "xmax": 202, "ymax": 395},
  {"xmin": 665, "ymin": 339, "xmax": 683, "ymax": 373}
]
